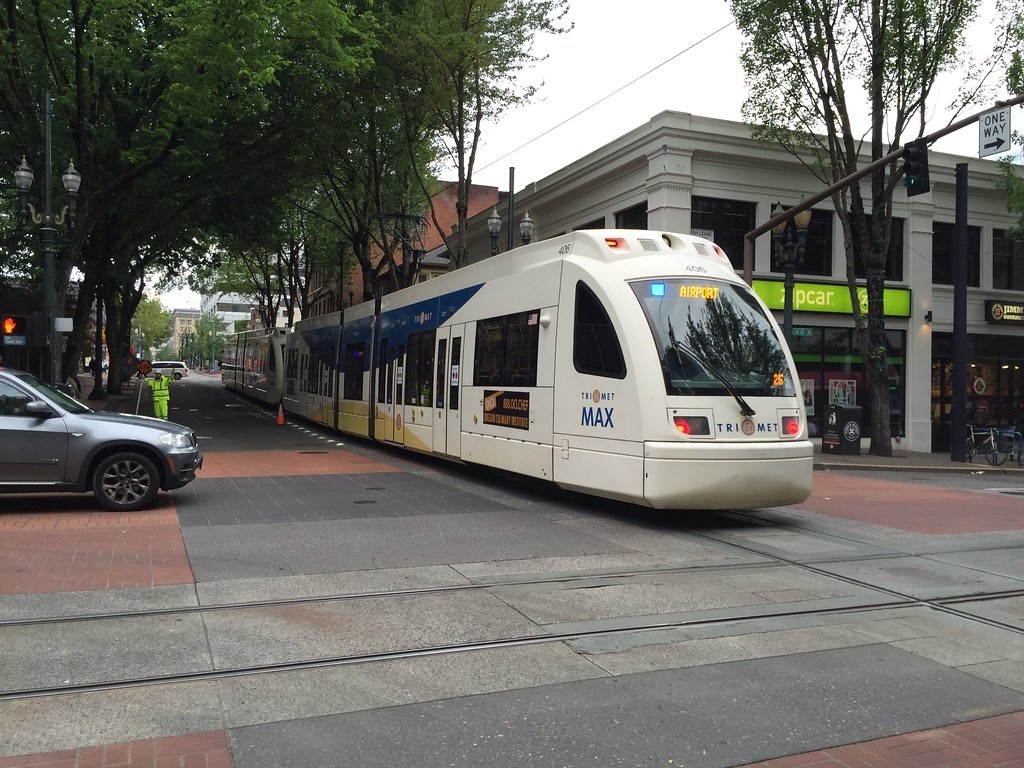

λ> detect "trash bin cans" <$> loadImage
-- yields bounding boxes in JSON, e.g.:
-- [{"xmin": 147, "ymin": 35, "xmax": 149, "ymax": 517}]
[
  {"xmin": 822, "ymin": 403, "xmax": 862, "ymax": 455},
  {"xmin": 55, "ymin": 382, "xmax": 71, "ymax": 396}
]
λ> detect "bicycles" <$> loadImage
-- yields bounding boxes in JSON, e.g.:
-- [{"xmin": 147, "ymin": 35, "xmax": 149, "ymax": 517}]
[{"xmin": 965, "ymin": 424, "xmax": 1010, "ymax": 466}]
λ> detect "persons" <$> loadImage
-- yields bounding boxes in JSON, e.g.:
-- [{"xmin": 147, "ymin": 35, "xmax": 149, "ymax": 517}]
[
  {"xmin": 140, "ymin": 369, "xmax": 175, "ymax": 420},
  {"xmin": 89, "ymin": 359, "xmax": 96, "ymax": 377},
  {"xmin": 66, "ymin": 372, "xmax": 81, "ymax": 401}
]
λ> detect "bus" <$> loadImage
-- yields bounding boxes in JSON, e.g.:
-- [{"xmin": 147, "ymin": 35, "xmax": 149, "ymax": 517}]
[{"xmin": 796, "ymin": 355, "xmax": 901, "ymax": 396}]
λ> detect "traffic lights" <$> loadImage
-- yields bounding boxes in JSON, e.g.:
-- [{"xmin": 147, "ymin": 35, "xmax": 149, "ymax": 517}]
[
  {"xmin": 899, "ymin": 140, "xmax": 932, "ymax": 196},
  {"xmin": 1, "ymin": 314, "xmax": 25, "ymax": 339}
]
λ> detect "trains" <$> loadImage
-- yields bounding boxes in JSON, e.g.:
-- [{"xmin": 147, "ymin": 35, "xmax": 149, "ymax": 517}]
[{"xmin": 217, "ymin": 229, "xmax": 815, "ymax": 510}]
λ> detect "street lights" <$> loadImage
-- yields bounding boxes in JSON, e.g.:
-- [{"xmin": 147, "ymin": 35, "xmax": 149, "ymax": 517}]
[
  {"xmin": 89, "ymin": 271, "xmax": 112, "ymax": 402},
  {"xmin": 770, "ymin": 197, "xmax": 812, "ymax": 352},
  {"xmin": 15, "ymin": 90, "xmax": 82, "ymax": 387},
  {"xmin": 485, "ymin": 165, "xmax": 536, "ymax": 382}
]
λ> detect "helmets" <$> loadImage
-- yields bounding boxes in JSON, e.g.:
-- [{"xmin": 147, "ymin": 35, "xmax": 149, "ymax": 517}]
[{"xmin": 155, "ymin": 369, "xmax": 162, "ymax": 374}]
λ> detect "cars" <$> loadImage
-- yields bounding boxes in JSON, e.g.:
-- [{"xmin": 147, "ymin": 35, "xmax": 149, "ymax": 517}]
[
  {"xmin": 139, "ymin": 362, "xmax": 190, "ymax": 380},
  {"xmin": 0, "ymin": 369, "xmax": 202, "ymax": 510}
]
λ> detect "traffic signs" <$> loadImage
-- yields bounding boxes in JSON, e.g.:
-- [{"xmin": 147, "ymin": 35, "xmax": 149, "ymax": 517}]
[{"xmin": 979, "ymin": 105, "xmax": 1012, "ymax": 160}]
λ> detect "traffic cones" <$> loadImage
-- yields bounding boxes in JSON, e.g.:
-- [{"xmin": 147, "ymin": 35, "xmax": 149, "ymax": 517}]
[{"xmin": 276, "ymin": 403, "xmax": 286, "ymax": 427}]
